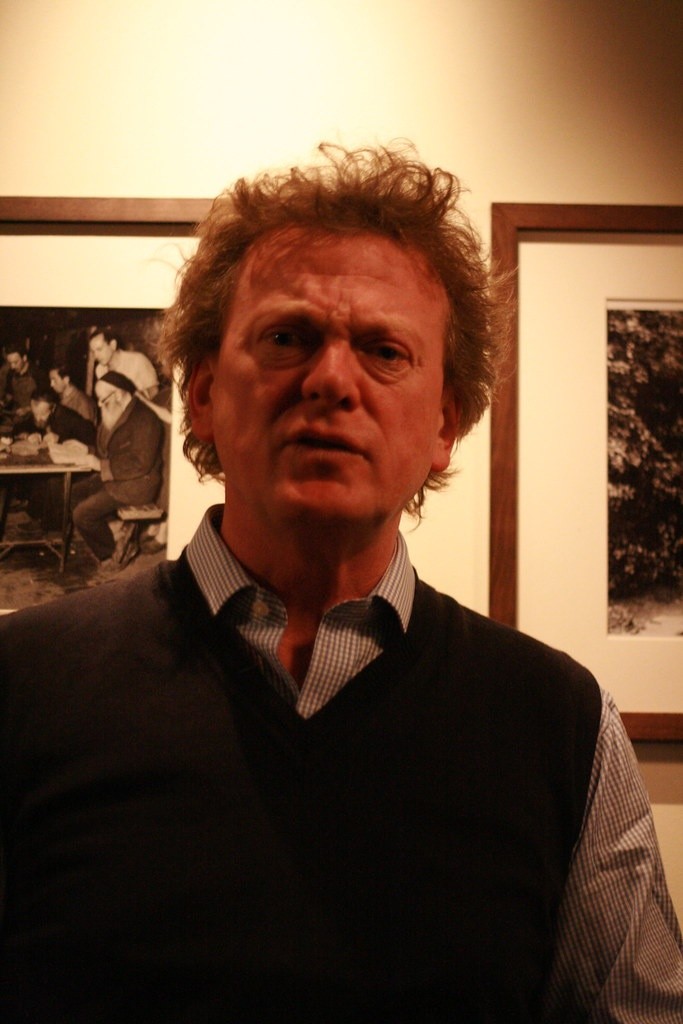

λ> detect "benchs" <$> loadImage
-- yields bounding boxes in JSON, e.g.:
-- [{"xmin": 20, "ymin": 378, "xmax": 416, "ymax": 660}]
[{"xmin": 114, "ymin": 503, "xmax": 163, "ymax": 563}]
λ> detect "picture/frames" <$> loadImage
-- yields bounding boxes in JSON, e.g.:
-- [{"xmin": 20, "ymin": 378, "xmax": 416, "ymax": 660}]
[
  {"xmin": 0, "ymin": 195, "xmax": 225, "ymax": 615},
  {"xmin": 490, "ymin": 200, "xmax": 683, "ymax": 746}
]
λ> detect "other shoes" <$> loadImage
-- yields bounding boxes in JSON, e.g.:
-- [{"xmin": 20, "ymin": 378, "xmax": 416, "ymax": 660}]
[{"xmin": 87, "ymin": 556, "xmax": 121, "ymax": 586}]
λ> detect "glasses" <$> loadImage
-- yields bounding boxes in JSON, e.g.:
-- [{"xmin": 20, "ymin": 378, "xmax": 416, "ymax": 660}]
[{"xmin": 101, "ymin": 392, "xmax": 115, "ymax": 405}]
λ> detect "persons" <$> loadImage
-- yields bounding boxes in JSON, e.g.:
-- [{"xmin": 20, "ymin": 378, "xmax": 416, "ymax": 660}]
[
  {"xmin": 0, "ymin": 149, "xmax": 683, "ymax": 1024},
  {"xmin": 0, "ymin": 328, "xmax": 171, "ymax": 575}
]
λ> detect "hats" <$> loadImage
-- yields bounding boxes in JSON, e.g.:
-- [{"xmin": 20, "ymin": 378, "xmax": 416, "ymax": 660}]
[{"xmin": 100, "ymin": 371, "xmax": 137, "ymax": 397}]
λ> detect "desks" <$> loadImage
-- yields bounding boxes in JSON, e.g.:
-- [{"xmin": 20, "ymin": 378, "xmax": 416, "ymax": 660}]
[{"xmin": 0, "ymin": 465, "xmax": 95, "ymax": 577}]
